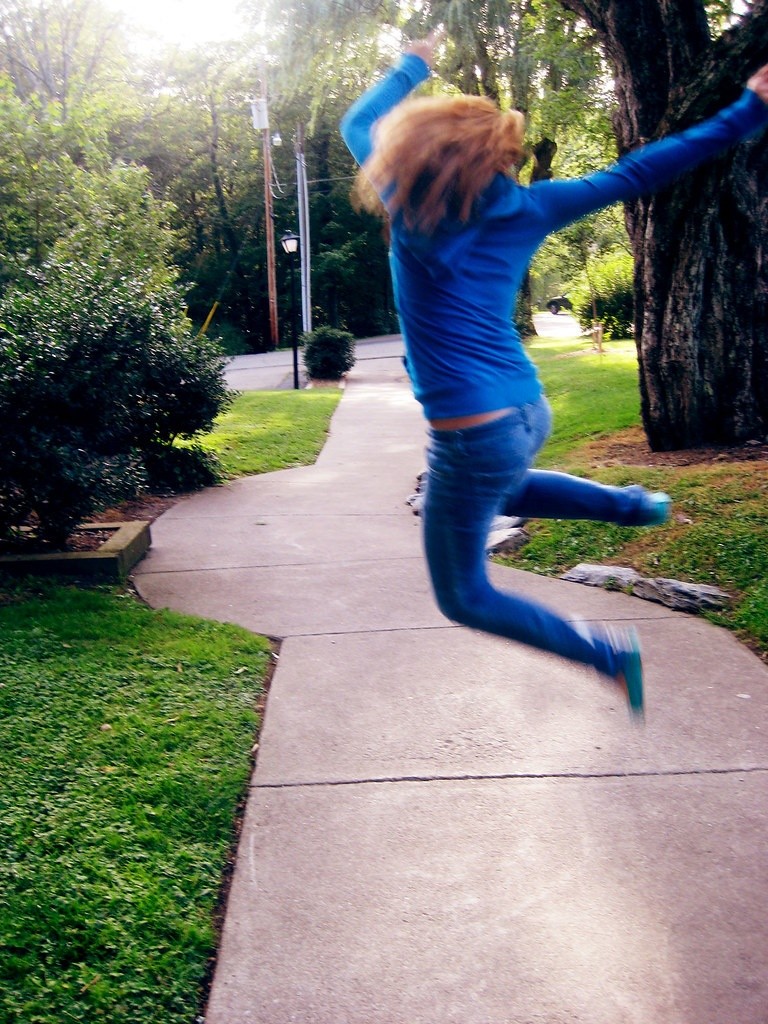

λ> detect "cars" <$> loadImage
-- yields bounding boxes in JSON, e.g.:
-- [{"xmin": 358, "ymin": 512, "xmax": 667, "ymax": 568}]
[{"xmin": 546, "ymin": 292, "xmax": 573, "ymax": 315}]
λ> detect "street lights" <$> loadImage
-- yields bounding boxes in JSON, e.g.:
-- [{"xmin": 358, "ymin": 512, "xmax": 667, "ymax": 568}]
[{"xmin": 251, "ymin": 96, "xmax": 281, "ymax": 349}]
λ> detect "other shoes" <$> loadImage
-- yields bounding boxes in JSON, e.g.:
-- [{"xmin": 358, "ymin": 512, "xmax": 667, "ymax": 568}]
[
  {"xmin": 647, "ymin": 492, "xmax": 672, "ymax": 527},
  {"xmin": 616, "ymin": 622, "xmax": 645, "ymax": 714}
]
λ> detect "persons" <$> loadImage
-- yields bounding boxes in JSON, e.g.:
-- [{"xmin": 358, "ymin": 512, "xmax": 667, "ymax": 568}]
[{"xmin": 341, "ymin": 31, "xmax": 768, "ymax": 728}]
